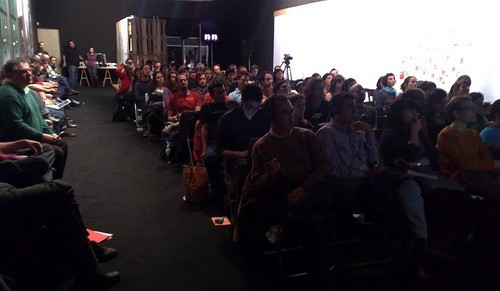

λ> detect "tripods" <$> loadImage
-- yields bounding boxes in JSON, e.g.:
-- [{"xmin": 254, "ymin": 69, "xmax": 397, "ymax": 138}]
[{"xmin": 282, "ymin": 63, "xmax": 292, "ymax": 81}]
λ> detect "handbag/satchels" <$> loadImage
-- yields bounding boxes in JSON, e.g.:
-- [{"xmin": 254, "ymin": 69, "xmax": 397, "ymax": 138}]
[{"xmin": 182, "ymin": 164, "xmax": 208, "ymax": 204}]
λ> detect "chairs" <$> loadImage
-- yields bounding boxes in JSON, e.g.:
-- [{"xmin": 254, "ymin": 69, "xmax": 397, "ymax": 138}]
[
  {"xmin": 234, "ymin": 137, "xmax": 393, "ymax": 279},
  {"xmin": 145, "ymin": 93, "xmax": 160, "ymax": 142}
]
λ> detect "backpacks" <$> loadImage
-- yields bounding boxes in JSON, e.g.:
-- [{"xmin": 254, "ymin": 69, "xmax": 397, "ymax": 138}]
[{"xmin": 113, "ymin": 104, "xmax": 128, "ymax": 121}]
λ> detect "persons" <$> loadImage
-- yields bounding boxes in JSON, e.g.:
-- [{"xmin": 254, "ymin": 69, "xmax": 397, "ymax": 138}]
[
  {"xmin": 0, "ymin": 51, "xmax": 119, "ymax": 291},
  {"xmin": 112, "ymin": 57, "xmax": 500, "ymax": 291},
  {"xmin": 85, "ymin": 46, "xmax": 102, "ymax": 87},
  {"xmin": 62, "ymin": 40, "xmax": 84, "ymax": 90},
  {"xmin": 34, "ymin": 41, "xmax": 48, "ymax": 54}
]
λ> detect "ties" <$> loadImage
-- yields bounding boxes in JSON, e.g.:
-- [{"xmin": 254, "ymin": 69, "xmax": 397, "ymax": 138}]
[{"xmin": 347, "ymin": 124, "xmax": 361, "ymax": 168}]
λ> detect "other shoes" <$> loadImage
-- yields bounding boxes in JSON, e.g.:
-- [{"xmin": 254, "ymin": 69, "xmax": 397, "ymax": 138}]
[
  {"xmin": 63, "ymin": 129, "xmax": 75, "ymax": 137},
  {"xmin": 90, "ymin": 240, "xmax": 116, "ymax": 262},
  {"xmin": 71, "ymin": 99, "xmax": 85, "ymax": 107},
  {"xmin": 68, "ymin": 90, "xmax": 79, "ymax": 95},
  {"xmin": 100, "ymin": 270, "xmax": 119, "ymax": 286}
]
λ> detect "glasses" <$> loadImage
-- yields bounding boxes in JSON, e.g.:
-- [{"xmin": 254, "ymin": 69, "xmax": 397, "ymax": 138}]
[
  {"xmin": 247, "ymin": 105, "xmax": 259, "ymax": 111},
  {"xmin": 274, "ymin": 108, "xmax": 296, "ymax": 116}
]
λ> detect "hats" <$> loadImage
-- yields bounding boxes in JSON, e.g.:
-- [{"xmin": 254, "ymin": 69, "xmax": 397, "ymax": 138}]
[
  {"xmin": 178, "ymin": 66, "xmax": 189, "ymax": 72},
  {"xmin": 386, "ymin": 88, "xmax": 427, "ymax": 123}
]
